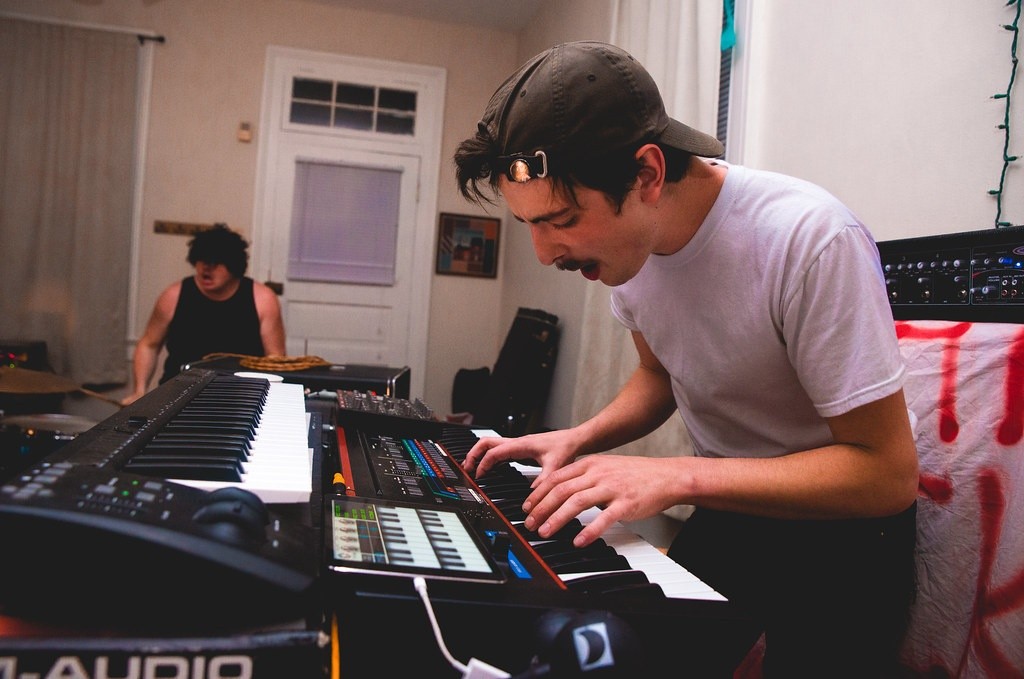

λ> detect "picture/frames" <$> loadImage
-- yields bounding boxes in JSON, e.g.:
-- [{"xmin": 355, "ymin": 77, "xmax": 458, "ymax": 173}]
[{"xmin": 435, "ymin": 211, "xmax": 503, "ymax": 280}]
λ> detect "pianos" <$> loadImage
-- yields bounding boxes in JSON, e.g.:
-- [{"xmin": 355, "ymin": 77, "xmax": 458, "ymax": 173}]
[{"xmin": 0, "ymin": 362, "xmax": 756, "ymax": 679}]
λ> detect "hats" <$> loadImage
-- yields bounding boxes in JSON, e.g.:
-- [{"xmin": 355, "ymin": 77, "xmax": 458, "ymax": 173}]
[{"xmin": 476, "ymin": 41, "xmax": 726, "ymax": 182}]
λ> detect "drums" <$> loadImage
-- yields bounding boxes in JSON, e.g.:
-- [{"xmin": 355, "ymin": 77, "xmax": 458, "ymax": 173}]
[{"xmin": 0, "ymin": 412, "xmax": 103, "ymax": 488}]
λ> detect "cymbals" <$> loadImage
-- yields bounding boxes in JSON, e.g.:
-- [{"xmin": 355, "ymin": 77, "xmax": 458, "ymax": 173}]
[{"xmin": 0, "ymin": 364, "xmax": 83, "ymax": 394}]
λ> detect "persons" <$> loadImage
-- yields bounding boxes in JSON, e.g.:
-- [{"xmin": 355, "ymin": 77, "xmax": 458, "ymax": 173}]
[
  {"xmin": 461, "ymin": 40, "xmax": 919, "ymax": 678},
  {"xmin": 122, "ymin": 221, "xmax": 286, "ymax": 404}
]
